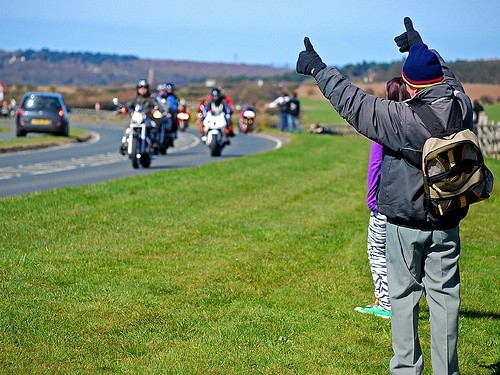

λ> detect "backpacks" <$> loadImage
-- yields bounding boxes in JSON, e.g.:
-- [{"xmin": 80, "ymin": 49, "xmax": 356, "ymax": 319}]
[{"xmin": 408, "ymin": 97, "xmax": 494, "ymax": 216}]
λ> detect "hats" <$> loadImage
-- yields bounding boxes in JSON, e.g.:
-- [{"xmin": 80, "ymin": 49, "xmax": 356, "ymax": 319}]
[{"xmin": 401, "ymin": 45, "xmax": 444, "ymax": 89}]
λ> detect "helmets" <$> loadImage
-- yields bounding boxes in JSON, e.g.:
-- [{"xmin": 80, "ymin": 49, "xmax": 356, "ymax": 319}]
[
  {"xmin": 136, "ymin": 78, "xmax": 149, "ymax": 88},
  {"xmin": 166, "ymin": 83, "xmax": 175, "ymax": 90},
  {"xmin": 212, "ymin": 88, "xmax": 220, "ymax": 97}
]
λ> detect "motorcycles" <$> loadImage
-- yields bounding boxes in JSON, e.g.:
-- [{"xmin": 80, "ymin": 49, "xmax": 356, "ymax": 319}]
[
  {"xmin": 155, "ymin": 100, "xmax": 178, "ymax": 154},
  {"xmin": 197, "ymin": 97, "xmax": 232, "ymax": 156},
  {"xmin": 111, "ymin": 94, "xmax": 158, "ymax": 170},
  {"xmin": 240, "ymin": 106, "xmax": 258, "ymax": 133}
]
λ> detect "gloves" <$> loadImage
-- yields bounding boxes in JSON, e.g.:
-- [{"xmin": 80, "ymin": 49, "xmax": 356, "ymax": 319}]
[
  {"xmin": 296, "ymin": 36, "xmax": 326, "ymax": 78},
  {"xmin": 395, "ymin": 17, "xmax": 426, "ymax": 52}
]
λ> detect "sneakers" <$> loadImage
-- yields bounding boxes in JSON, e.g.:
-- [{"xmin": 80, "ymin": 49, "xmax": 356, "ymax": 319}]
[{"xmin": 354, "ymin": 299, "xmax": 392, "ymax": 319}]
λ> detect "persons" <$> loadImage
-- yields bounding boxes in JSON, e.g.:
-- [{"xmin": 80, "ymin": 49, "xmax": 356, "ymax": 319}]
[
  {"xmin": 274, "ymin": 92, "xmax": 342, "ymax": 135},
  {"xmin": 116, "ymin": 79, "xmax": 164, "ymax": 155},
  {"xmin": 297, "ymin": 17, "xmax": 473, "ymax": 375},
  {"xmin": 8, "ymin": 96, "xmax": 16, "ymax": 110},
  {"xmin": 354, "ymin": 74, "xmax": 413, "ymax": 318},
  {"xmin": 473, "ymin": 99, "xmax": 483, "ymax": 135},
  {"xmin": 202, "ymin": 88, "xmax": 233, "ymax": 141},
  {"xmin": 150, "ymin": 81, "xmax": 255, "ymax": 156}
]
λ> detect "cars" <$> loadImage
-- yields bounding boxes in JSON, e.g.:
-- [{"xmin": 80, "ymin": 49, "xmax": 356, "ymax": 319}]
[{"xmin": 14, "ymin": 91, "xmax": 71, "ymax": 138}]
